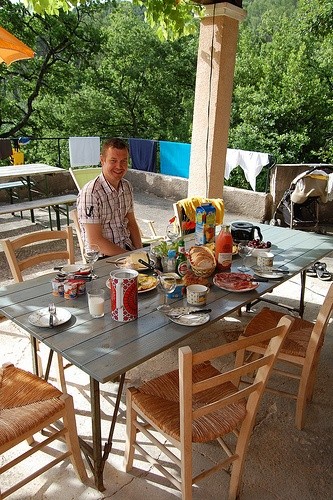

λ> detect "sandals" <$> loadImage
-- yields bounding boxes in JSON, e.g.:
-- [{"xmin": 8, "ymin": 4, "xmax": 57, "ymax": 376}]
[
  {"xmin": 304, "ymin": 266, "xmax": 317, "ymax": 276},
  {"xmin": 314, "ymin": 261, "xmax": 332, "ymax": 281}
]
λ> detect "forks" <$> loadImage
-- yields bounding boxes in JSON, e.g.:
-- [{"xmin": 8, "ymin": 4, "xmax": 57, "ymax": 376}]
[
  {"xmin": 166, "ymin": 308, "xmax": 212, "ymax": 319},
  {"xmin": 48, "ymin": 303, "xmax": 56, "ymax": 329},
  {"xmin": 250, "ymin": 266, "xmax": 288, "ymax": 273}
]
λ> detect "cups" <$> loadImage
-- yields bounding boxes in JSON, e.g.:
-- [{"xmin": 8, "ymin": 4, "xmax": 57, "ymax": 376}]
[
  {"xmin": 186, "ymin": 285, "xmax": 210, "ymax": 306},
  {"xmin": 165, "ymin": 224, "xmax": 180, "ymax": 246},
  {"xmin": 62, "ymin": 265, "xmax": 80, "ymax": 274},
  {"xmin": 87, "ymin": 288, "xmax": 105, "ymax": 318},
  {"xmin": 256, "ymin": 252, "xmax": 274, "ymax": 268},
  {"xmin": 160, "ymin": 257, "xmax": 177, "ymax": 272},
  {"xmin": 109, "ymin": 269, "xmax": 139, "ymax": 322}
]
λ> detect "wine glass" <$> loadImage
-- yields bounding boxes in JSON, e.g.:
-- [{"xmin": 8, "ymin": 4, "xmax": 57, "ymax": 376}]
[
  {"xmin": 157, "ymin": 275, "xmax": 176, "ymax": 312},
  {"xmin": 237, "ymin": 243, "xmax": 254, "ymax": 272},
  {"xmin": 83, "ymin": 244, "xmax": 100, "ymax": 280}
]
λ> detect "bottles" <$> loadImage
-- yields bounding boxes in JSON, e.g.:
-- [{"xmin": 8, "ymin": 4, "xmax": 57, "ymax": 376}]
[
  {"xmin": 215, "ymin": 224, "xmax": 233, "ymax": 271},
  {"xmin": 175, "ymin": 239, "xmax": 188, "ymax": 278}
]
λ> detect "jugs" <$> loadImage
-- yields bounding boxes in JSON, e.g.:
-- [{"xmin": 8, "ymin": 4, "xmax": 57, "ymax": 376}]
[{"xmin": 231, "ymin": 221, "xmax": 264, "ymax": 245}]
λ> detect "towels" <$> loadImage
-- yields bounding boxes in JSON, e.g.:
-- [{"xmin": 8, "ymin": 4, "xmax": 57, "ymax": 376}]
[
  {"xmin": 224, "ymin": 148, "xmax": 269, "ymax": 192},
  {"xmin": 128, "ymin": 138, "xmax": 157, "ymax": 172},
  {"xmin": 159, "ymin": 141, "xmax": 191, "ymax": 179},
  {"xmin": 68, "ymin": 136, "xmax": 101, "ymax": 167}
]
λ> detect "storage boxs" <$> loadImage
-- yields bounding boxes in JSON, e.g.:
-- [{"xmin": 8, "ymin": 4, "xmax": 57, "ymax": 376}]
[
  {"xmin": 195, "ymin": 202, "xmax": 216, "ymax": 246},
  {"xmin": 231, "ymin": 221, "xmax": 256, "ymax": 245}
]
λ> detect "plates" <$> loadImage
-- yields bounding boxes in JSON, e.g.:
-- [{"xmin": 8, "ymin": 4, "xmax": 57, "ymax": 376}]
[
  {"xmin": 252, "ymin": 266, "xmax": 285, "ymax": 278},
  {"xmin": 212, "ymin": 277, "xmax": 260, "ymax": 292},
  {"xmin": 114, "ymin": 257, "xmax": 156, "ymax": 271},
  {"xmin": 106, "ymin": 274, "xmax": 157, "ymax": 294},
  {"xmin": 164, "ymin": 306, "xmax": 210, "ymax": 326},
  {"xmin": 28, "ymin": 307, "xmax": 72, "ymax": 327}
]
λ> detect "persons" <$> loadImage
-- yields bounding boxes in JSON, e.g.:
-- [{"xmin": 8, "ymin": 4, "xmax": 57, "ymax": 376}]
[{"xmin": 76, "ymin": 138, "xmax": 151, "ymax": 264}]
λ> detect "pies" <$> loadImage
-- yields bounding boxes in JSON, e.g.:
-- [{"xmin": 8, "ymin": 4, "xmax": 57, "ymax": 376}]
[{"xmin": 138, "ymin": 274, "xmax": 157, "ymax": 290}]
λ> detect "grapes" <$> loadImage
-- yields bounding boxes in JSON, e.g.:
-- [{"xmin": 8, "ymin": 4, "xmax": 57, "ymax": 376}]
[{"xmin": 248, "ymin": 239, "xmax": 266, "ymax": 249}]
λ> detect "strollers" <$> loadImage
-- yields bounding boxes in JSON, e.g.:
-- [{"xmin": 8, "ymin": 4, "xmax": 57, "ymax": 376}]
[{"xmin": 270, "ymin": 165, "xmax": 333, "ymax": 235}]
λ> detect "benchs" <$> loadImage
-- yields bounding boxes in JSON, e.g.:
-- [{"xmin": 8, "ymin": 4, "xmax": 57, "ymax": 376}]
[
  {"xmin": 0, "ymin": 193, "xmax": 78, "ymax": 215},
  {"xmin": 0, "ymin": 180, "xmax": 35, "ymax": 190}
]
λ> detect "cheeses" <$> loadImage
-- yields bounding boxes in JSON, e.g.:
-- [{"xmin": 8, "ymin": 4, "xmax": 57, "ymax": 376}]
[{"xmin": 126, "ymin": 252, "xmax": 149, "ymax": 268}]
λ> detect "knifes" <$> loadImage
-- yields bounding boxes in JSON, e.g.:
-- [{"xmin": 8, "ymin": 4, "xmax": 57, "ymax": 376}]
[{"xmin": 138, "ymin": 259, "xmax": 164, "ymax": 276}]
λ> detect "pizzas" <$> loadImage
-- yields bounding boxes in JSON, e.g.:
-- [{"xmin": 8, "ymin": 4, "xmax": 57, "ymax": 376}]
[{"xmin": 215, "ymin": 273, "xmax": 254, "ymax": 290}]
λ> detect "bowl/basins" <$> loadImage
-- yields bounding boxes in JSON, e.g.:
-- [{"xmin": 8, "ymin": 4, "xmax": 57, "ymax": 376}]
[
  {"xmin": 245, "ymin": 244, "xmax": 271, "ymax": 257},
  {"xmin": 160, "ymin": 273, "xmax": 185, "ymax": 297}
]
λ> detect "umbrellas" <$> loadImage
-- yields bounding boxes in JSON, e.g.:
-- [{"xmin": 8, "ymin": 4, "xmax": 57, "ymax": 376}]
[{"xmin": 0, "ymin": 26, "xmax": 35, "ymax": 66}]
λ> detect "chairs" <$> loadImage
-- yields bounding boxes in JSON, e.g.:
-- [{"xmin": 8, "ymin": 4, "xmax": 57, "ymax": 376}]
[
  {"xmin": 122, "ymin": 312, "xmax": 297, "ymax": 500},
  {"xmin": 2, "ymin": 227, "xmax": 75, "ymax": 378},
  {"xmin": 135, "ymin": 217, "xmax": 166, "ymax": 244},
  {"xmin": 234, "ymin": 282, "xmax": 333, "ymax": 431},
  {"xmin": 0, "ymin": 361, "xmax": 89, "ymax": 500},
  {"xmin": 71, "ymin": 208, "xmax": 87, "ymax": 264}
]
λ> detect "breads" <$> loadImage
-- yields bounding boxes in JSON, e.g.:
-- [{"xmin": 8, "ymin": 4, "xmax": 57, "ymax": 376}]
[
  {"xmin": 190, "ymin": 247, "xmax": 214, "ymax": 267},
  {"xmin": 183, "ymin": 272, "xmax": 208, "ymax": 286}
]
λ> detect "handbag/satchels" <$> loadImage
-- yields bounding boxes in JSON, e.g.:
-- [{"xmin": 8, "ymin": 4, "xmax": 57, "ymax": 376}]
[{"xmin": 231, "ymin": 222, "xmax": 263, "ymax": 243}]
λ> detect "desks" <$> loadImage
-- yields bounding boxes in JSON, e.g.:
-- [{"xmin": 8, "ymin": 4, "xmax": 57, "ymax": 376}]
[
  {"xmin": 0, "ymin": 162, "xmax": 67, "ymax": 223},
  {"xmin": 0, "ymin": 218, "xmax": 333, "ymax": 493}
]
